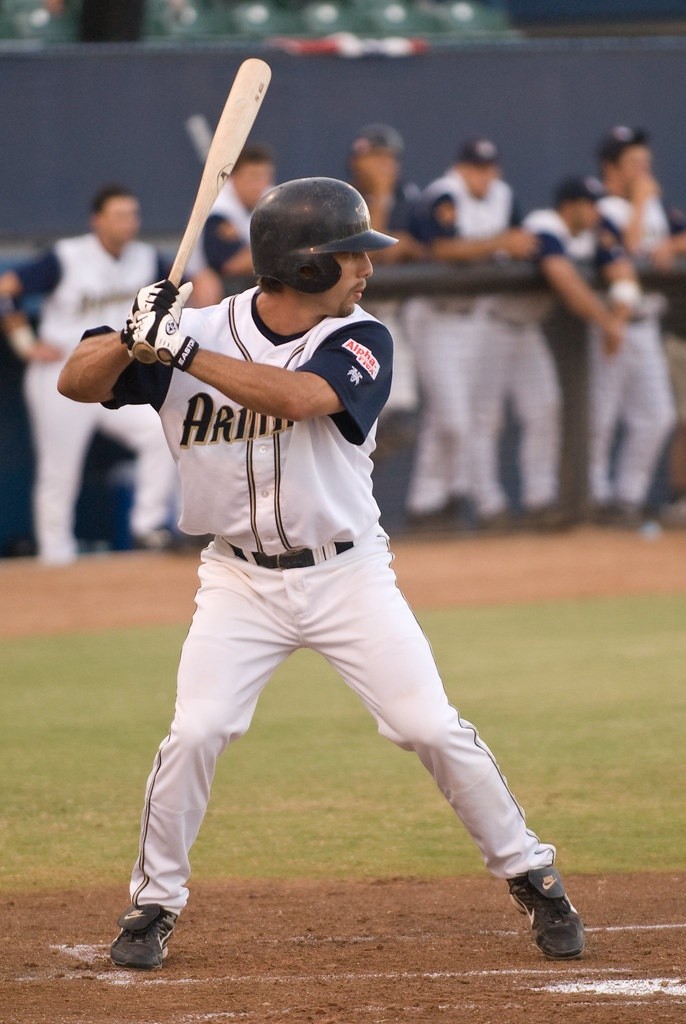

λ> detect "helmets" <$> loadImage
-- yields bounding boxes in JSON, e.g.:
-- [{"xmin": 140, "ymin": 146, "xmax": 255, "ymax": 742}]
[{"xmin": 250, "ymin": 177, "xmax": 399, "ymax": 295}]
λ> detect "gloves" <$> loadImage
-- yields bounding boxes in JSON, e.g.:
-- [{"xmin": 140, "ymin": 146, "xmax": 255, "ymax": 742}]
[
  {"xmin": 119, "ymin": 309, "xmax": 199, "ymax": 372},
  {"xmin": 128, "ymin": 278, "xmax": 193, "ymax": 329}
]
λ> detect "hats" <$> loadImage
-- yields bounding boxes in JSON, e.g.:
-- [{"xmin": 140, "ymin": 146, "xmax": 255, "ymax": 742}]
[
  {"xmin": 601, "ymin": 122, "xmax": 651, "ymax": 158},
  {"xmin": 459, "ymin": 137, "xmax": 505, "ymax": 166},
  {"xmin": 553, "ymin": 170, "xmax": 609, "ymax": 202},
  {"xmin": 233, "ymin": 139, "xmax": 281, "ymax": 164},
  {"xmin": 353, "ymin": 122, "xmax": 408, "ymax": 155}
]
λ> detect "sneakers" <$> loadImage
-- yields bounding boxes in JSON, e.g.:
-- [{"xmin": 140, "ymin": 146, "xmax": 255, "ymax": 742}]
[
  {"xmin": 109, "ymin": 904, "xmax": 179, "ymax": 970},
  {"xmin": 505, "ymin": 866, "xmax": 586, "ymax": 962}
]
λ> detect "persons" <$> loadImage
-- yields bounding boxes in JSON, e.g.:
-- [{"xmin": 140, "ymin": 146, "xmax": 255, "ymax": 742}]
[
  {"xmin": 189, "ymin": 146, "xmax": 276, "ymax": 309},
  {"xmin": 0, "ymin": 185, "xmax": 179, "ymax": 563},
  {"xmin": 57, "ymin": 176, "xmax": 585, "ymax": 970},
  {"xmin": 348, "ymin": 126, "xmax": 686, "ymax": 529}
]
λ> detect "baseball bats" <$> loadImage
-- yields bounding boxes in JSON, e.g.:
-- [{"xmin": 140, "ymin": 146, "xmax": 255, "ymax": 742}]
[{"xmin": 133, "ymin": 56, "xmax": 272, "ymax": 364}]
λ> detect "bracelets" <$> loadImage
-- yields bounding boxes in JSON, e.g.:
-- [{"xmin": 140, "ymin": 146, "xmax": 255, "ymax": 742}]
[{"xmin": 9, "ymin": 325, "xmax": 37, "ymax": 352}]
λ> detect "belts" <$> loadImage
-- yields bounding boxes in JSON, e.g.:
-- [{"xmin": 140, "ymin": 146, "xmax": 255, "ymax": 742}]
[{"xmin": 232, "ymin": 538, "xmax": 354, "ymax": 569}]
